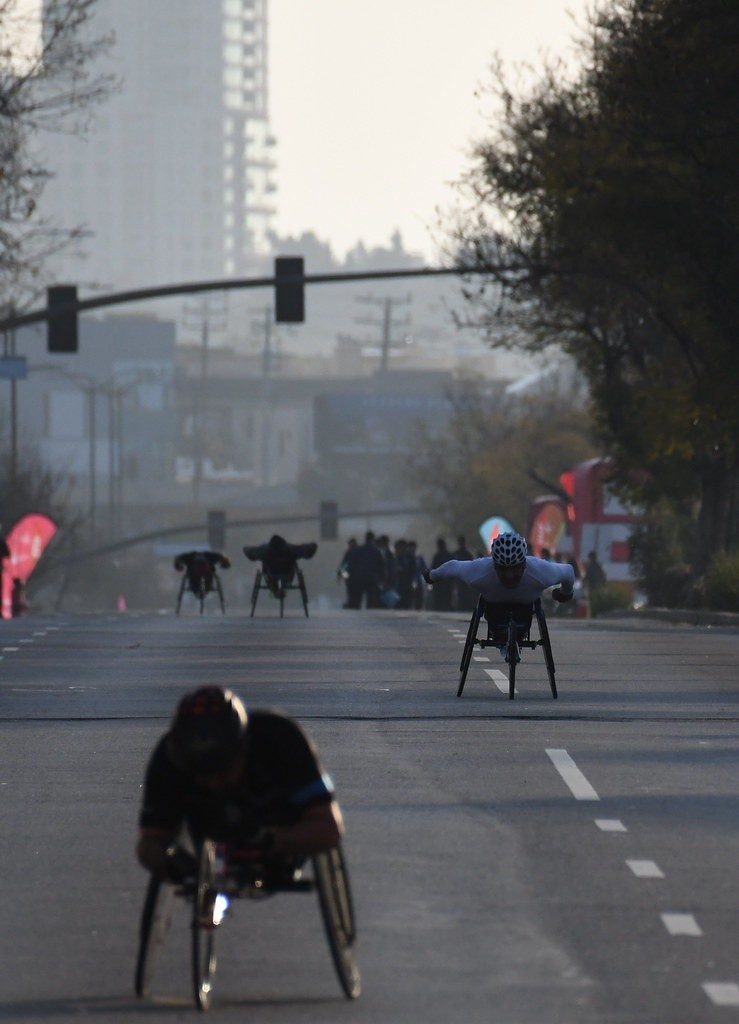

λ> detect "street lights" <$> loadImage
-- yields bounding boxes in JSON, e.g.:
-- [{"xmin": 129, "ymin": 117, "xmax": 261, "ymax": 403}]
[
  {"xmin": 0, "ymin": 225, "xmax": 100, "ymax": 529},
  {"xmin": 28, "ymin": 362, "xmax": 167, "ymax": 583}
]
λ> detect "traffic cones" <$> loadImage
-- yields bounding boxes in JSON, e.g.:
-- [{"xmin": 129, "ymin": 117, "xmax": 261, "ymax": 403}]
[{"xmin": 574, "ymin": 583, "xmax": 590, "ymax": 620}]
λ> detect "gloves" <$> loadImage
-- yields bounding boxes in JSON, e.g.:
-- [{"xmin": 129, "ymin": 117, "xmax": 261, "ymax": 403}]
[
  {"xmin": 552, "ymin": 587, "xmax": 574, "ymax": 604},
  {"xmin": 421, "ymin": 568, "xmax": 433, "ymax": 585}
]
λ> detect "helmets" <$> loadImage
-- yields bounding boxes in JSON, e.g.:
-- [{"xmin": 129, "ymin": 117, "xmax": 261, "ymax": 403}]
[
  {"xmin": 491, "ymin": 531, "xmax": 527, "ymax": 566},
  {"xmin": 172, "ymin": 683, "xmax": 248, "ymax": 762}
]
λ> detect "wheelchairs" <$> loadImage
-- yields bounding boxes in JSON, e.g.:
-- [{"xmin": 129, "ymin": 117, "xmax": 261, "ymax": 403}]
[
  {"xmin": 175, "ymin": 571, "xmax": 226, "ymax": 618},
  {"xmin": 250, "ymin": 561, "xmax": 311, "ymax": 621},
  {"xmin": 132, "ymin": 831, "xmax": 363, "ymax": 1014},
  {"xmin": 456, "ymin": 594, "xmax": 559, "ymax": 702}
]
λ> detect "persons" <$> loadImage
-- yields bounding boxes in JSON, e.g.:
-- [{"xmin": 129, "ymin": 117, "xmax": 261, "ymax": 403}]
[
  {"xmin": 245, "ymin": 535, "xmax": 318, "ymax": 597},
  {"xmin": 339, "ymin": 532, "xmax": 607, "ymax": 642},
  {"xmin": 172, "ymin": 552, "xmax": 230, "ymax": 595},
  {"xmin": 134, "ymin": 685, "xmax": 344, "ymax": 889}
]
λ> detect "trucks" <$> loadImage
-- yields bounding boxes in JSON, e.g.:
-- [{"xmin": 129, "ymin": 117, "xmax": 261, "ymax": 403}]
[{"xmin": 526, "ymin": 453, "xmax": 669, "ymax": 603}]
